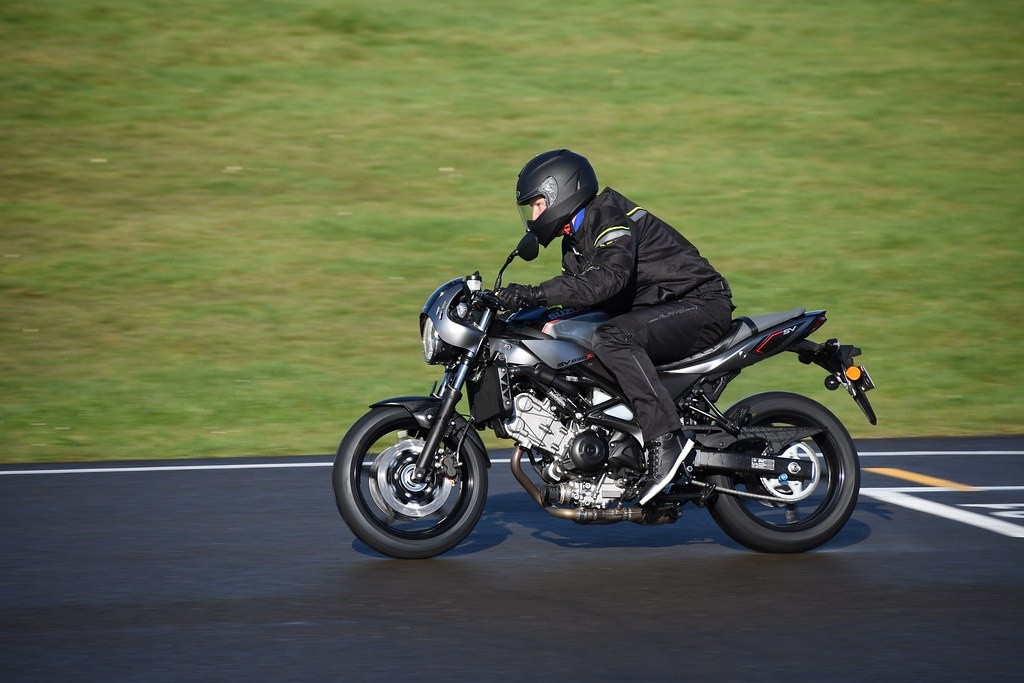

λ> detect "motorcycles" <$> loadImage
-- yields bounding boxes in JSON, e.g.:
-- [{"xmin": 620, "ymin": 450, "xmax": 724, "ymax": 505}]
[{"xmin": 333, "ymin": 230, "xmax": 878, "ymax": 562}]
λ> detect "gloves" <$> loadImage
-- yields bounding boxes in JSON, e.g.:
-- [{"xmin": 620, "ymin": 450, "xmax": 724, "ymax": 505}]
[{"xmin": 499, "ymin": 282, "xmax": 547, "ymax": 313}]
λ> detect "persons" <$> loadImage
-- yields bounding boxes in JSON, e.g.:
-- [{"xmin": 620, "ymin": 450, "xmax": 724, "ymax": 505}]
[{"xmin": 493, "ymin": 149, "xmax": 732, "ymax": 505}]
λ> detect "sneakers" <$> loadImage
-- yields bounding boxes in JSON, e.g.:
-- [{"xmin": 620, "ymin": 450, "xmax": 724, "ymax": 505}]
[{"xmin": 639, "ymin": 429, "xmax": 695, "ymax": 505}]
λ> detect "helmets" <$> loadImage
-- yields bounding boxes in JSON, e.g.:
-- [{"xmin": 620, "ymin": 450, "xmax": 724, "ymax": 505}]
[{"xmin": 515, "ymin": 148, "xmax": 599, "ymax": 249}]
